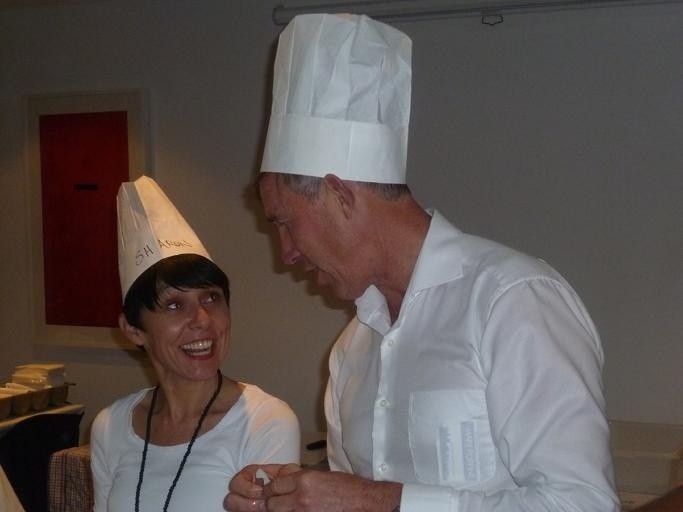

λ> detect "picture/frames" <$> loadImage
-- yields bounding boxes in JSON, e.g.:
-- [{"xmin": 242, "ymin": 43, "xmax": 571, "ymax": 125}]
[{"xmin": 22, "ymin": 88, "xmax": 147, "ymax": 353}]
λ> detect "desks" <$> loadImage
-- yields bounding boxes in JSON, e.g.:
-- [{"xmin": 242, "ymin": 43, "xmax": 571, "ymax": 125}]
[{"xmin": 46, "ymin": 431, "xmax": 330, "ymax": 512}]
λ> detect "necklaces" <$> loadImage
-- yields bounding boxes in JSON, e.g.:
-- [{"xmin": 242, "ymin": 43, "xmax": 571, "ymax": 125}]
[{"xmin": 134, "ymin": 368, "xmax": 222, "ymax": 512}]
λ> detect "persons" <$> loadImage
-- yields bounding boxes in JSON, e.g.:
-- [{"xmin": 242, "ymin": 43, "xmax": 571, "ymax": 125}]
[
  {"xmin": 219, "ymin": 171, "xmax": 625, "ymax": 512},
  {"xmin": 86, "ymin": 254, "xmax": 302, "ymax": 511}
]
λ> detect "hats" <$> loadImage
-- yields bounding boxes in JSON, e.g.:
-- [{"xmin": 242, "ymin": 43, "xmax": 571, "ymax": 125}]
[
  {"xmin": 259, "ymin": 11, "xmax": 414, "ymax": 186},
  {"xmin": 115, "ymin": 175, "xmax": 214, "ymax": 304}
]
